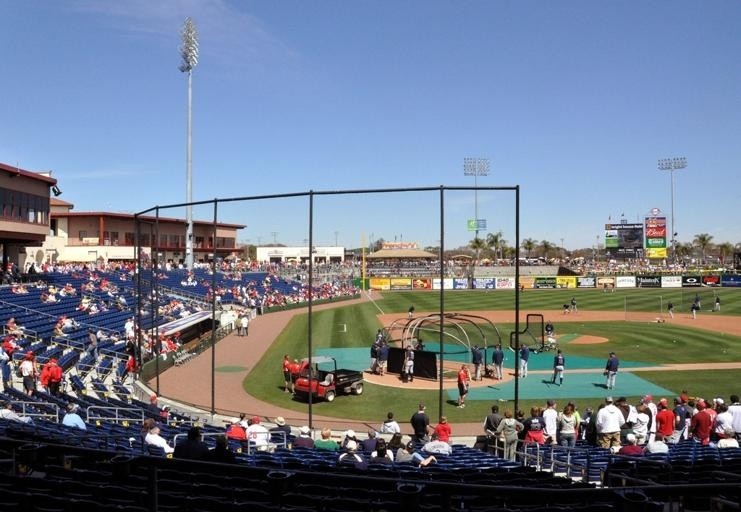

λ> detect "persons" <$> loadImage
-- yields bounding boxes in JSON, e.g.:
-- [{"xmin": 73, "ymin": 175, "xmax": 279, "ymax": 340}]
[
  {"xmin": 2, "ymin": 261, "xmax": 151, "ymax": 314},
  {"xmin": 545, "ymin": 321, "xmax": 554, "ymax": 343},
  {"xmin": 236, "ymin": 315, "xmax": 249, "ymax": 336},
  {"xmin": 606, "ymin": 352, "xmax": 618, "ymax": 389},
  {"xmin": 149, "ymin": 288, "xmax": 203, "ymax": 319},
  {"xmin": 227, "ymin": 404, "xmax": 452, "ymax": 466},
  {"xmin": 153, "ymin": 261, "xmax": 741, "ymax": 286},
  {"xmin": 519, "ymin": 344, "xmax": 529, "ymax": 378},
  {"xmin": 492, "ymin": 345, "xmax": 504, "ymax": 380},
  {"xmin": 0, "ymin": 400, "xmax": 32, "ymax": 423},
  {"xmin": 668, "ymin": 300, "xmax": 673, "ymax": 318},
  {"xmin": 403, "ymin": 339, "xmax": 423, "ymax": 382},
  {"xmin": 458, "ymin": 365, "xmax": 468, "ymax": 407},
  {"xmin": 692, "ymin": 294, "xmax": 701, "ymax": 318},
  {"xmin": 371, "ymin": 340, "xmax": 388, "ymax": 375},
  {"xmin": 55, "ymin": 314, "xmax": 80, "ymax": 336},
  {"xmin": 8, "ymin": 318, "xmax": 21, "ymax": 338},
  {"xmin": 89, "ymin": 317, "xmax": 183, "ymax": 374},
  {"xmin": 283, "ymin": 354, "xmax": 300, "ymax": 392},
  {"xmin": 408, "ymin": 305, "xmax": 413, "ymax": 317},
  {"xmin": 207, "ymin": 275, "xmax": 358, "ymax": 314},
  {"xmin": 62, "ymin": 404, "xmax": 86, "ymax": 428},
  {"xmin": 472, "ymin": 345, "xmax": 483, "ymax": 379},
  {"xmin": 484, "ymin": 390, "xmax": 741, "ymax": 456},
  {"xmin": 553, "ymin": 350, "xmax": 565, "ymax": 385},
  {"xmin": 143, "ymin": 419, "xmax": 235, "ymax": 464},
  {"xmin": 716, "ymin": 296, "xmax": 720, "ymax": 310},
  {"xmin": 563, "ymin": 298, "xmax": 576, "ymax": 314},
  {"xmin": 0, "ymin": 336, "xmax": 62, "ymax": 396}
]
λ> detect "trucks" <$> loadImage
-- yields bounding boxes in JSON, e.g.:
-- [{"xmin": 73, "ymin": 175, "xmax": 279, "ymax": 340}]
[{"xmin": 295, "ymin": 356, "xmax": 365, "ymax": 403}]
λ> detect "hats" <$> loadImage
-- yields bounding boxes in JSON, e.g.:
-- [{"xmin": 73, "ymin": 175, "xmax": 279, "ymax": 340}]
[
  {"xmin": 298, "ymin": 425, "xmax": 310, "ymax": 434},
  {"xmin": 605, "ymin": 395, "xmax": 725, "ymax": 441},
  {"xmin": 252, "ymin": 415, "xmax": 260, "ymax": 424},
  {"xmin": 70, "ymin": 403, "xmax": 79, "ymax": 412},
  {"xmin": 345, "ymin": 430, "xmax": 355, "ymax": 437},
  {"xmin": 400, "ymin": 436, "xmax": 416, "ymax": 449},
  {"xmin": 26, "ymin": 351, "xmax": 57, "ymax": 367},
  {"xmin": 274, "ymin": 417, "xmax": 285, "ymax": 426}
]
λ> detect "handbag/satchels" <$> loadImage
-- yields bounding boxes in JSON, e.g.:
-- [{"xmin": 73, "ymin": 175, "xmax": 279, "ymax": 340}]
[{"xmin": 16, "ymin": 369, "xmax": 22, "ymax": 379}]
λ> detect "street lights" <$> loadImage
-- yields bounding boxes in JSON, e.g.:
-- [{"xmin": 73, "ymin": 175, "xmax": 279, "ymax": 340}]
[
  {"xmin": 656, "ymin": 155, "xmax": 689, "ymax": 266},
  {"xmin": 463, "ymin": 156, "xmax": 490, "ymax": 266},
  {"xmin": 333, "ymin": 229, "xmax": 339, "ymax": 246},
  {"xmin": 176, "ymin": 15, "xmax": 199, "ymax": 273},
  {"xmin": 560, "ymin": 237, "xmax": 564, "ymax": 259}
]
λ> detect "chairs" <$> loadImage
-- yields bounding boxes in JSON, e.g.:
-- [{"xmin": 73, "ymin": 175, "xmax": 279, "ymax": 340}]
[{"xmin": 0, "ymin": 261, "xmax": 741, "ymax": 512}]
